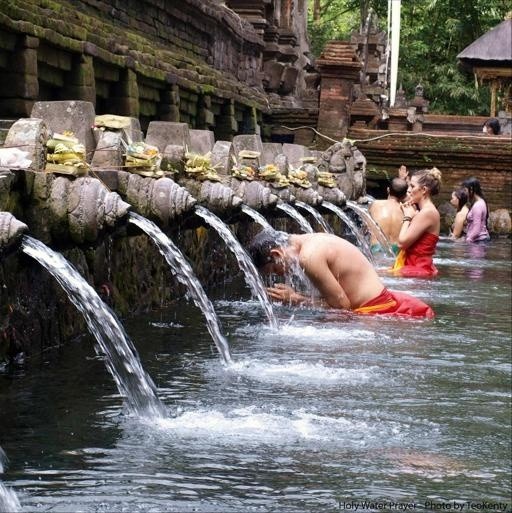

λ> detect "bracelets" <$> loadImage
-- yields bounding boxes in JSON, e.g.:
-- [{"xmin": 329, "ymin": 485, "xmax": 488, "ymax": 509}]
[{"xmin": 400, "ymin": 215, "xmax": 412, "ymax": 224}]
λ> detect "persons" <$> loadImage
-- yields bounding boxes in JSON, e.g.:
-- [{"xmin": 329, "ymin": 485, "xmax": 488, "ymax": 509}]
[
  {"xmin": 362, "ymin": 176, "xmax": 405, "ymax": 264},
  {"xmin": 449, "ymin": 244, "xmax": 466, "ymax": 277},
  {"xmin": 447, "ymin": 187, "xmax": 472, "ymax": 240},
  {"xmin": 482, "ymin": 115, "xmax": 502, "ymax": 135},
  {"xmin": 396, "ymin": 162, "xmax": 420, "ymax": 211},
  {"xmin": 386, "ymin": 166, "xmax": 445, "ymax": 279},
  {"xmin": 465, "ymin": 244, "xmax": 487, "ymax": 280},
  {"xmin": 460, "ymin": 174, "xmax": 493, "ymax": 243},
  {"xmin": 244, "ymin": 225, "xmax": 438, "ymax": 321}
]
